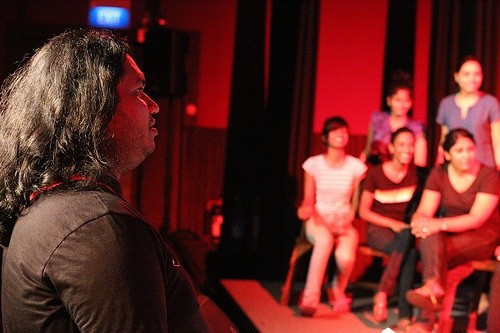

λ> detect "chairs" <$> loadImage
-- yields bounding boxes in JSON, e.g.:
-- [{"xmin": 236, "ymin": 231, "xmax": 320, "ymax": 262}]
[{"xmin": 280, "ymin": 230, "xmax": 500, "ymax": 333}]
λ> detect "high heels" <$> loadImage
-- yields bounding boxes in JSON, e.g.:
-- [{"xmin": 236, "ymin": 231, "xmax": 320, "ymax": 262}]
[
  {"xmin": 327, "ymin": 282, "xmax": 350, "ymax": 312},
  {"xmin": 297, "ymin": 285, "xmax": 321, "ymax": 316}
]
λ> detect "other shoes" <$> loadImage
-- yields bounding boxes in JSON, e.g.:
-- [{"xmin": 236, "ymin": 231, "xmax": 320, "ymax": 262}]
[
  {"xmin": 388, "ymin": 318, "xmax": 432, "ymax": 333},
  {"xmin": 372, "ymin": 289, "xmax": 392, "ymax": 324},
  {"xmin": 405, "ymin": 274, "xmax": 444, "ymax": 310}
]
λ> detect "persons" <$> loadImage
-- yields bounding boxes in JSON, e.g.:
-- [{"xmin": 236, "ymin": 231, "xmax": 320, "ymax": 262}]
[
  {"xmin": 0, "ymin": 30, "xmax": 213, "ymax": 333},
  {"xmin": 433, "ymin": 56, "xmax": 500, "ymax": 171},
  {"xmin": 402, "ymin": 128, "xmax": 500, "ymax": 333},
  {"xmin": 358, "ymin": 127, "xmax": 423, "ymax": 333},
  {"xmin": 298, "ymin": 115, "xmax": 369, "ymax": 315},
  {"xmin": 358, "ymin": 83, "xmax": 428, "ymax": 181}
]
